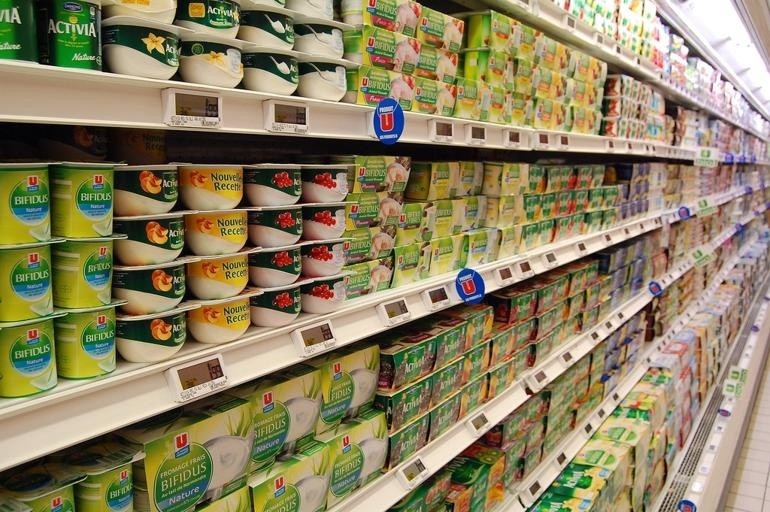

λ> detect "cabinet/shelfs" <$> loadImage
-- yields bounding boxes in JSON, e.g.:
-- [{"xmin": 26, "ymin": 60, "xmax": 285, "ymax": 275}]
[{"xmin": 0, "ymin": 0, "xmax": 770, "ymax": 512}]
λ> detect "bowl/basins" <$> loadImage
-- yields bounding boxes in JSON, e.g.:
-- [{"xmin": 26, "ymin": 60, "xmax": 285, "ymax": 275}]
[
  {"xmin": 241, "ymin": 10, "xmax": 294, "ymax": 49},
  {"xmin": 302, "ymin": 242, "xmax": 343, "ymax": 277},
  {"xmin": 183, "ymin": 36, "xmax": 244, "ymax": 87},
  {"xmin": 116, "ymin": 311, "xmax": 186, "ymax": 362},
  {"xmin": 245, "ymin": 169, "xmax": 302, "ymax": 205},
  {"xmin": 303, "ymin": 207, "xmax": 345, "ymax": 239},
  {"xmin": 103, "ymin": 15, "xmax": 182, "ymax": 79},
  {"xmin": 302, "ymin": 278, "xmax": 345, "ymax": 313},
  {"xmin": 185, "ymin": 212, "xmax": 248, "ymax": 254},
  {"xmin": 249, "ymin": 208, "xmax": 303, "ymax": 247},
  {"xmin": 296, "ymin": 23, "xmax": 344, "ymax": 57},
  {"xmin": 288, "ymin": 1, "xmax": 334, "ymax": 18},
  {"xmin": 188, "ymin": 299, "xmax": 251, "ymax": 343},
  {"xmin": 113, "ymin": 261, "xmax": 185, "ymax": 314},
  {"xmin": 114, "ymin": 166, "xmax": 178, "ymax": 213},
  {"xmin": 113, "ymin": 215, "xmax": 184, "ymax": 265},
  {"xmin": 102, "ymin": 0, "xmax": 177, "ymax": 24},
  {"xmin": 242, "ymin": 1, "xmax": 283, "ymax": 8},
  {"xmin": 250, "ymin": 248, "xmax": 302, "ymax": 288},
  {"xmin": 242, "ymin": 52, "xmax": 299, "ymax": 93},
  {"xmin": 303, "ymin": 169, "xmax": 347, "ymax": 202},
  {"xmin": 252, "ymin": 288, "xmax": 301, "ymax": 327},
  {"xmin": 187, "ymin": 255, "xmax": 249, "ymax": 298},
  {"xmin": 179, "ymin": 166, "xmax": 244, "ymax": 210},
  {"xmin": 296, "ymin": 62, "xmax": 348, "ymax": 101},
  {"xmin": 179, "ymin": 0, "xmax": 240, "ymax": 38}
]
